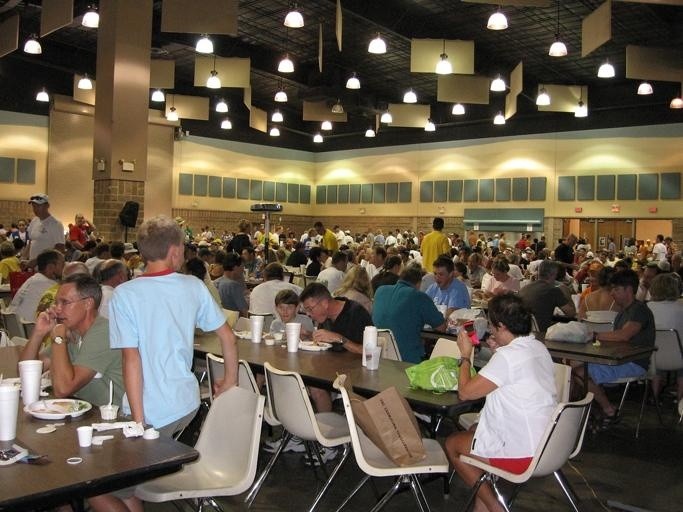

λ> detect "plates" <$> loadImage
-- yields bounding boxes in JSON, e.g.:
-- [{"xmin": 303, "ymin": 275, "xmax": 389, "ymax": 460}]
[
  {"xmin": 0, "ymin": 377, "xmax": 52, "ymax": 393},
  {"xmin": 299, "ymin": 341, "xmax": 332, "ymax": 352},
  {"xmin": 24, "ymin": 398, "xmax": 92, "ymax": 420}
]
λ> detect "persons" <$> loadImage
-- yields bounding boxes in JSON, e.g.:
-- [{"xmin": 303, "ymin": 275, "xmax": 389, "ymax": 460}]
[
  {"xmin": 172, "ymin": 217, "xmax": 683, "ymax": 466},
  {"xmin": 17, "ymin": 273, "xmax": 127, "ymax": 512},
  {"xmin": 85, "ymin": 215, "xmax": 239, "ymax": 511},
  {"xmin": 444, "ymin": 293, "xmax": 556, "ymax": 512},
  {"xmin": 1, "ymin": 192, "xmax": 146, "ymax": 351}
]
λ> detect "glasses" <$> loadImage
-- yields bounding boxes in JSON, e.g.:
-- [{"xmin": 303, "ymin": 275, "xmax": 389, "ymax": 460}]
[{"xmin": 303, "ymin": 297, "xmax": 325, "ymax": 313}]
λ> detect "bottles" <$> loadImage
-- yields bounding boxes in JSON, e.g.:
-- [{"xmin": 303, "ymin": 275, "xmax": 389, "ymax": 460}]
[{"xmin": 360, "ymin": 325, "xmax": 376, "ymax": 367}]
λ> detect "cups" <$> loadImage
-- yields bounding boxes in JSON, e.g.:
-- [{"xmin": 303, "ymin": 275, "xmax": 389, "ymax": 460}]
[
  {"xmin": 243, "ymin": 269, "xmax": 248, "ymax": 279},
  {"xmin": 435, "ymin": 304, "xmax": 446, "ymax": 318},
  {"xmin": 100, "ymin": 405, "xmax": 118, "ymax": 419},
  {"xmin": 364, "ymin": 344, "xmax": 381, "ymax": 368},
  {"xmin": 299, "ymin": 264, "xmax": 304, "ymax": 273},
  {"xmin": 249, "ymin": 315, "xmax": 265, "ymax": 343},
  {"xmin": 261, "ymin": 332, "xmax": 282, "ymax": 345},
  {"xmin": 468, "ymin": 288, "xmax": 473, "ymax": 300},
  {"xmin": 582, "ymin": 284, "xmax": 589, "ymax": 292},
  {"xmin": 285, "ymin": 322, "xmax": 302, "ymax": 351},
  {"xmin": 76, "ymin": 425, "xmax": 92, "ymax": 447},
  {"xmin": 17, "ymin": 360, "xmax": 47, "ymax": 404},
  {"xmin": 0, "ymin": 386, "xmax": 20, "ymax": 442}
]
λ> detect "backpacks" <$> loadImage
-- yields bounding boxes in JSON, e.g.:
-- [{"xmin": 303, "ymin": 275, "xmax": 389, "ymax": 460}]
[{"xmin": 404, "ymin": 355, "xmax": 477, "ymax": 395}]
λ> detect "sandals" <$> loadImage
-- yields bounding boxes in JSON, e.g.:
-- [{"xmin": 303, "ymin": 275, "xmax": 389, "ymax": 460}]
[{"xmin": 594, "ymin": 408, "xmax": 623, "ymax": 434}]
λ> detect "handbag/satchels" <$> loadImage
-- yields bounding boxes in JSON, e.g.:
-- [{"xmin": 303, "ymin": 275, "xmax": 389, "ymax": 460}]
[
  {"xmin": 344, "ymin": 373, "xmax": 428, "ymax": 467},
  {"xmin": 8, "ymin": 264, "xmax": 36, "ymax": 300}
]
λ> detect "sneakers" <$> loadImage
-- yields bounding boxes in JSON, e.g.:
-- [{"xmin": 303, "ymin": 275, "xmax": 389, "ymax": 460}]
[
  {"xmin": 263, "ymin": 433, "xmax": 307, "ymax": 453},
  {"xmin": 304, "ymin": 441, "xmax": 339, "ymax": 468},
  {"xmin": 647, "ymin": 397, "xmax": 663, "ymax": 407}
]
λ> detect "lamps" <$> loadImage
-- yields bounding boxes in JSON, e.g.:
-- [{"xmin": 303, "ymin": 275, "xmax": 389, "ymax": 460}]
[
  {"xmin": 118, "ymin": 157, "xmax": 137, "ymax": 171},
  {"xmin": 94, "ymin": 156, "xmax": 106, "ymax": 172}
]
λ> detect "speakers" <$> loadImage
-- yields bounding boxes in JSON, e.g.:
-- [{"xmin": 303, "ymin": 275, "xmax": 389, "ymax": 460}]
[{"xmin": 119, "ymin": 201, "xmax": 138, "ymax": 227}]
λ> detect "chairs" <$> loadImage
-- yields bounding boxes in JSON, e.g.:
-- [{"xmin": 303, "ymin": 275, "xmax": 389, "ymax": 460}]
[
  {"xmin": 246, "ymin": 311, "xmax": 276, "ymax": 331},
  {"xmin": 133, "ymin": 385, "xmax": 268, "ymax": 511},
  {"xmin": 0, "ymin": 282, "xmax": 47, "ymax": 350},
  {"xmin": 303, "ymin": 275, "xmax": 317, "ymax": 289},
  {"xmin": 173, "ymin": 382, "xmax": 208, "ymax": 443},
  {"xmin": 461, "ymin": 361, "xmax": 581, "ymax": 508},
  {"xmin": 335, "ymin": 374, "xmax": 453, "ymax": 512},
  {"xmin": 198, "ymin": 308, "xmax": 241, "ymax": 383},
  {"xmin": 246, "ymin": 359, "xmax": 352, "ymax": 512},
  {"xmin": 205, "ymin": 352, "xmax": 321, "ymax": 505},
  {"xmin": 458, "ymin": 390, "xmax": 597, "ymax": 512},
  {"xmin": 375, "ymin": 329, "xmax": 404, "ymax": 361}
]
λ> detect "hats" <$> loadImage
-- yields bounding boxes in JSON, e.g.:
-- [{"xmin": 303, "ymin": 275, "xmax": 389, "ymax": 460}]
[
  {"xmin": 576, "ymin": 243, "xmax": 586, "ymax": 251},
  {"xmin": 587, "ymin": 251, "xmax": 593, "ymax": 258},
  {"xmin": 190, "ymin": 236, "xmax": 212, "ymax": 246},
  {"xmin": 174, "ymin": 216, "xmax": 185, "ymax": 225},
  {"xmin": 124, "ymin": 243, "xmax": 138, "ymax": 254},
  {"xmin": 213, "ymin": 238, "xmax": 222, "ymax": 244},
  {"xmin": 254, "ymin": 244, "xmax": 265, "ymax": 252},
  {"xmin": 28, "ymin": 194, "xmax": 49, "ymax": 205},
  {"xmin": 618, "ymin": 251, "xmax": 642, "ymax": 256},
  {"xmin": 526, "ymin": 249, "xmax": 532, "ymax": 254},
  {"xmin": 657, "ymin": 260, "xmax": 670, "ymax": 271},
  {"xmin": 450, "ymin": 232, "xmax": 456, "ymax": 236},
  {"xmin": 344, "ymin": 230, "xmax": 350, "ymax": 234},
  {"xmin": 494, "ymin": 234, "xmax": 500, "ymax": 238}
]
